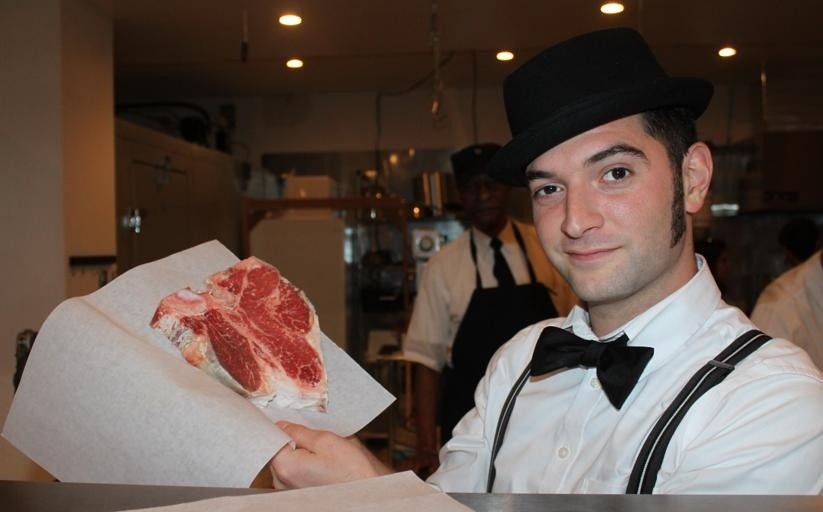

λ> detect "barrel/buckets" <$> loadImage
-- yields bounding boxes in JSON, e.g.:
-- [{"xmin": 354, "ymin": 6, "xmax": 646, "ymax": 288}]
[{"xmin": 412, "ymin": 171, "xmax": 454, "ymax": 218}]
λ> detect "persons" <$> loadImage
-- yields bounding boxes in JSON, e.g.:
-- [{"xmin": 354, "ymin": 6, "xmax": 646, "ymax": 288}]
[
  {"xmin": 403, "ymin": 144, "xmax": 579, "ymax": 479},
  {"xmin": 750, "ymin": 249, "xmax": 823, "ymax": 374},
  {"xmin": 270, "ymin": 28, "xmax": 822, "ymax": 496}
]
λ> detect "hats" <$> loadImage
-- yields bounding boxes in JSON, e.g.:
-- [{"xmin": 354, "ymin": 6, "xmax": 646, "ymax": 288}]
[
  {"xmin": 484, "ymin": 27, "xmax": 714, "ymax": 187},
  {"xmin": 449, "ymin": 143, "xmax": 503, "ymax": 180}
]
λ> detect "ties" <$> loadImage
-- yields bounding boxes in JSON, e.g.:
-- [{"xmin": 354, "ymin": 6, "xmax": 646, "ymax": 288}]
[{"xmin": 491, "ymin": 240, "xmax": 517, "ymax": 287}]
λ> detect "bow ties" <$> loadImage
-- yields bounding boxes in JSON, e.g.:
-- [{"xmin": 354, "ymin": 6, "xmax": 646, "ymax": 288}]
[{"xmin": 531, "ymin": 327, "xmax": 654, "ymax": 409}]
